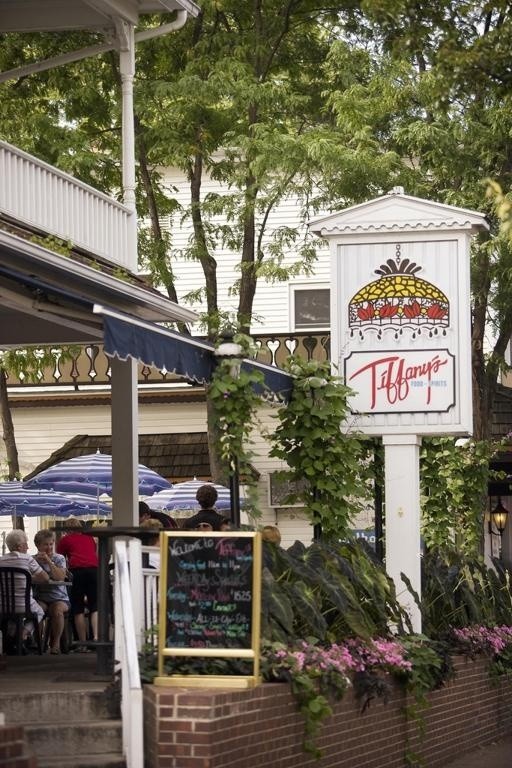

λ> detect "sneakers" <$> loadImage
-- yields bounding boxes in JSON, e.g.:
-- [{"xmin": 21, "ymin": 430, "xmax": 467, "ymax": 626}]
[{"xmin": 9, "ymin": 641, "xmax": 107, "ymax": 656}]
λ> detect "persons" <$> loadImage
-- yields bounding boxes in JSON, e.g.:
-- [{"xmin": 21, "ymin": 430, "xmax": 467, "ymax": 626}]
[
  {"xmin": 1, "ymin": 502, "xmax": 181, "ymax": 656},
  {"xmin": 263, "ymin": 526, "xmax": 281, "ymax": 546},
  {"xmin": 182, "ymin": 485, "xmax": 226, "ymax": 531}
]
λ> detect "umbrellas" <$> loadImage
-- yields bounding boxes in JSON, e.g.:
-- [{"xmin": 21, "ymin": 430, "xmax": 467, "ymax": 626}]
[
  {"xmin": 0, "ymin": 477, "xmax": 90, "ymax": 530},
  {"xmin": 53, "ymin": 491, "xmax": 112, "ymax": 553},
  {"xmin": 142, "ymin": 474, "xmax": 252, "ymax": 513},
  {"xmin": 24, "ymin": 447, "xmax": 174, "ymax": 525}
]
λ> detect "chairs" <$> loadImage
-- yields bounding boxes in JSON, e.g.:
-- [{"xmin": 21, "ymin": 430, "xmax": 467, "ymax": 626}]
[
  {"xmin": 108, "ymin": 562, "xmax": 159, "ymax": 642},
  {"xmin": 0, "ymin": 566, "xmax": 44, "ymax": 656},
  {"xmin": 23, "ymin": 612, "xmax": 71, "ymax": 654}
]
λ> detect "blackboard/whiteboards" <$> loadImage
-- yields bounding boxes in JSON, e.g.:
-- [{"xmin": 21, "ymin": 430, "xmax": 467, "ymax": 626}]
[
  {"xmin": 267, "ymin": 469, "xmax": 329, "ymax": 509},
  {"xmin": 339, "ymin": 530, "xmax": 377, "ymax": 553},
  {"xmin": 158, "ymin": 531, "xmax": 262, "ymax": 658}
]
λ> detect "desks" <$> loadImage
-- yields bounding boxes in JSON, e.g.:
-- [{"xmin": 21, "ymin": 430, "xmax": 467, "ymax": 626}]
[{"xmin": 30, "ymin": 580, "xmax": 73, "ymax": 586}]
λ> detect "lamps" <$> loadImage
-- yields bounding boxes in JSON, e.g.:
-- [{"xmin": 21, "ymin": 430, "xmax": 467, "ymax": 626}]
[{"xmin": 488, "ymin": 492, "xmax": 509, "ymax": 537}]
